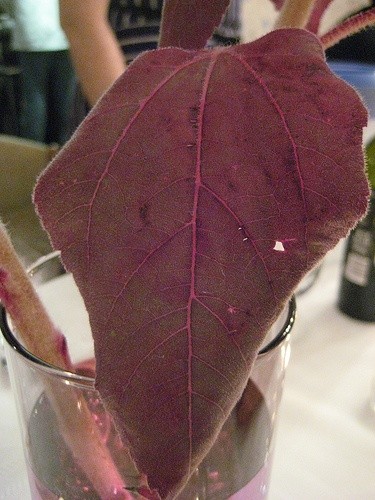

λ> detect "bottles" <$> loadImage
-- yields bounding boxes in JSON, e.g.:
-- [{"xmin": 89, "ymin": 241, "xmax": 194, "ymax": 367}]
[{"xmin": 337, "ymin": 137, "xmax": 375, "ymax": 322}]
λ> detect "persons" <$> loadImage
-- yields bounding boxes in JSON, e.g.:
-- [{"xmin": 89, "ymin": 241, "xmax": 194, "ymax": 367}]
[
  {"xmin": 60, "ymin": 1, "xmax": 240, "ymax": 134},
  {"xmin": 0, "ymin": 0, "xmax": 80, "ymax": 147}
]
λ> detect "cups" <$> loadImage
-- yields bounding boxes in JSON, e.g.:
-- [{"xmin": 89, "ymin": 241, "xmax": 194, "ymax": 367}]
[{"xmin": 0, "ymin": 251, "xmax": 296, "ymax": 500}]
